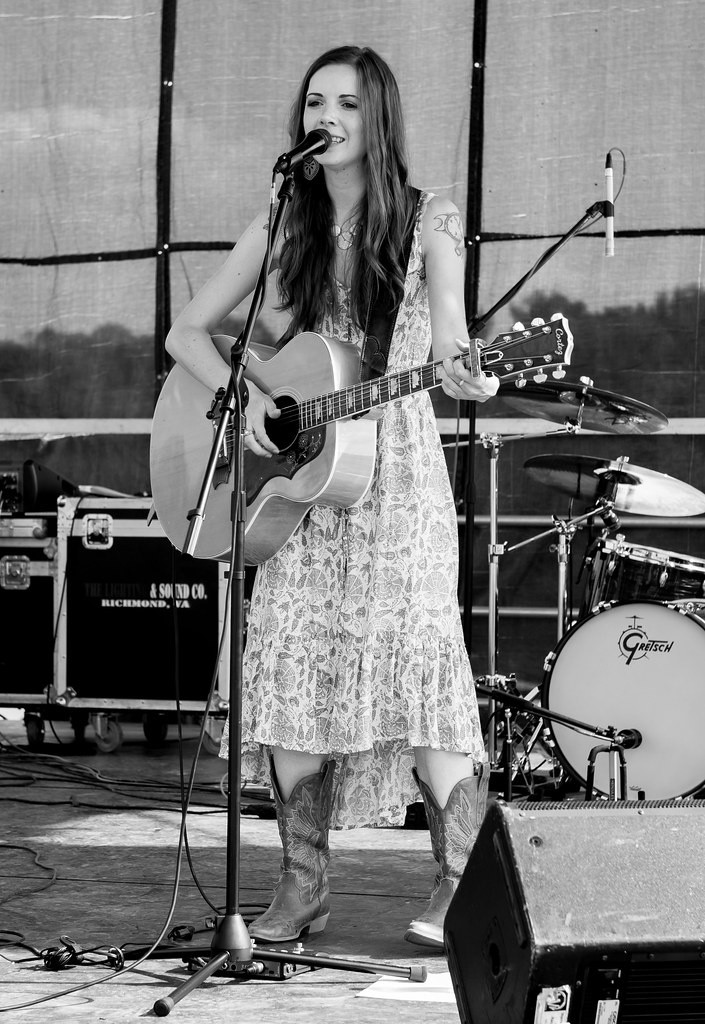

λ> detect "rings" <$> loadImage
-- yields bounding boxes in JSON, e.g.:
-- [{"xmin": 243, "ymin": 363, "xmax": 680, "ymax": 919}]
[
  {"xmin": 243, "ymin": 430, "xmax": 254, "ymax": 436},
  {"xmin": 457, "ymin": 380, "xmax": 464, "ymax": 387}
]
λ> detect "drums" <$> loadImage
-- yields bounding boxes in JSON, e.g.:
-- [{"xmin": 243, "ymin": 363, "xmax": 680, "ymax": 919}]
[
  {"xmin": 583, "ymin": 537, "xmax": 704, "ymax": 606},
  {"xmin": 538, "ymin": 605, "xmax": 705, "ymax": 799}
]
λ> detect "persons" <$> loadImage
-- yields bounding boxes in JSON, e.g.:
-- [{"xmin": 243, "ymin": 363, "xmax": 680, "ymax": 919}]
[{"xmin": 162, "ymin": 44, "xmax": 500, "ymax": 944}]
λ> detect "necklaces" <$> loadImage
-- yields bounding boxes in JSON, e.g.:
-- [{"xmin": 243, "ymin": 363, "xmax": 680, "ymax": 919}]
[{"xmin": 329, "ymin": 223, "xmax": 361, "ymax": 250}]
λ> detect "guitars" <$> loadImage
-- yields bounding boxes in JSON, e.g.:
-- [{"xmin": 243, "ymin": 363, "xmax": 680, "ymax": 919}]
[{"xmin": 146, "ymin": 311, "xmax": 577, "ymax": 566}]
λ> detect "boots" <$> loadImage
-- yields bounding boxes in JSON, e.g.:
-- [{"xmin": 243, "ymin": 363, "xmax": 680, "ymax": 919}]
[
  {"xmin": 404, "ymin": 762, "xmax": 490, "ymax": 947},
  {"xmin": 247, "ymin": 752, "xmax": 335, "ymax": 943}
]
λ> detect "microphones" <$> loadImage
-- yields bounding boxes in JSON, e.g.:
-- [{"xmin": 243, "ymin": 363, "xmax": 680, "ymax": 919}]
[
  {"xmin": 595, "ymin": 498, "xmax": 620, "ymax": 532},
  {"xmin": 612, "ymin": 728, "xmax": 642, "ymax": 750},
  {"xmin": 604, "ymin": 153, "xmax": 614, "ymax": 259},
  {"xmin": 272, "ymin": 129, "xmax": 332, "ymax": 175}
]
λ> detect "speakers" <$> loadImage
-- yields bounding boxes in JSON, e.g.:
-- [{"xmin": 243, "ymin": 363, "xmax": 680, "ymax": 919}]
[{"xmin": 442, "ymin": 799, "xmax": 705, "ymax": 1023}]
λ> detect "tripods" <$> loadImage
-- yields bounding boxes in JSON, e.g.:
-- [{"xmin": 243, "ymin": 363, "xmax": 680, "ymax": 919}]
[
  {"xmin": 443, "ymin": 404, "xmax": 585, "ymax": 769},
  {"xmin": 106, "ymin": 173, "xmax": 429, "ymax": 1017}
]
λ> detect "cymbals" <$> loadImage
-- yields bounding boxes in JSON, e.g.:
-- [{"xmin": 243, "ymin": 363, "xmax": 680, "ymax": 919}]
[
  {"xmin": 494, "ymin": 380, "xmax": 670, "ymax": 439},
  {"xmin": 523, "ymin": 451, "xmax": 704, "ymax": 520}
]
